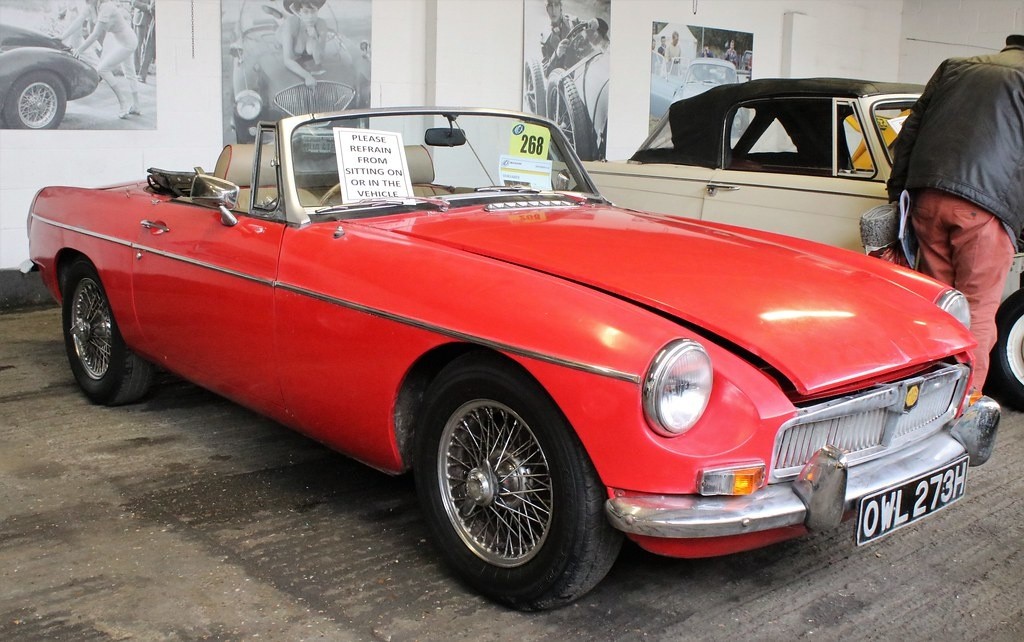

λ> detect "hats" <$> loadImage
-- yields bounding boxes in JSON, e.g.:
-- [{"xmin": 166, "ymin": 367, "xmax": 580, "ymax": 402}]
[
  {"xmin": 596, "ymin": 18, "xmax": 610, "ymax": 41},
  {"xmin": 1006, "ymin": 33, "xmax": 1024, "ymax": 46},
  {"xmin": 283, "ymin": 0, "xmax": 326, "ymax": 15}
]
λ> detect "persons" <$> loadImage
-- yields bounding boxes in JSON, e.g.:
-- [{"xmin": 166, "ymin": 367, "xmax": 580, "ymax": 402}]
[
  {"xmin": 886, "ymin": 35, "xmax": 1024, "ymax": 414},
  {"xmin": 262, "ymin": 0, "xmax": 328, "ymax": 88},
  {"xmin": 652, "ymin": 32, "xmax": 738, "ymax": 67},
  {"xmin": 38, "ymin": 0, "xmax": 155, "ymax": 120},
  {"xmin": 541, "ymin": 0, "xmax": 609, "ymax": 78}
]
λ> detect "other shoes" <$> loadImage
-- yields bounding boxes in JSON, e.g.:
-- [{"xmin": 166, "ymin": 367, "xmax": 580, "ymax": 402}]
[
  {"xmin": 118, "ymin": 112, "xmax": 128, "ymax": 118},
  {"xmin": 262, "ymin": 5, "xmax": 282, "ymax": 19},
  {"xmin": 129, "ymin": 106, "xmax": 142, "ymax": 115}
]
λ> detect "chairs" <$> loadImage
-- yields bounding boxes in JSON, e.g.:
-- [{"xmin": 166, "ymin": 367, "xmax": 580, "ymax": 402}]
[
  {"xmin": 213, "ymin": 143, "xmax": 319, "ymax": 218},
  {"xmin": 404, "ymin": 144, "xmax": 458, "ymax": 198}
]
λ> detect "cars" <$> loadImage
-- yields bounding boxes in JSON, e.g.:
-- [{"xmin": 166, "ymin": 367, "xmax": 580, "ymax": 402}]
[{"xmin": 650, "ymin": 50, "xmax": 753, "ymax": 127}]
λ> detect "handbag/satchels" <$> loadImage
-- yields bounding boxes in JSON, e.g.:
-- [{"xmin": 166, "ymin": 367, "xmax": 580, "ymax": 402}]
[{"xmin": 860, "ymin": 205, "xmax": 919, "ymax": 272}]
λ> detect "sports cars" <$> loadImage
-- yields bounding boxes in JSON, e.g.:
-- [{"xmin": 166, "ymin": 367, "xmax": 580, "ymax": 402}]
[
  {"xmin": 26, "ymin": 97, "xmax": 1003, "ymax": 617},
  {"xmin": 1, "ymin": 24, "xmax": 103, "ymax": 130},
  {"xmin": 526, "ymin": 21, "xmax": 609, "ymax": 162},
  {"xmin": 547, "ymin": 75, "xmax": 1023, "ymax": 415},
  {"xmin": 228, "ymin": 0, "xmax": 372, "ymax": 157}
]
